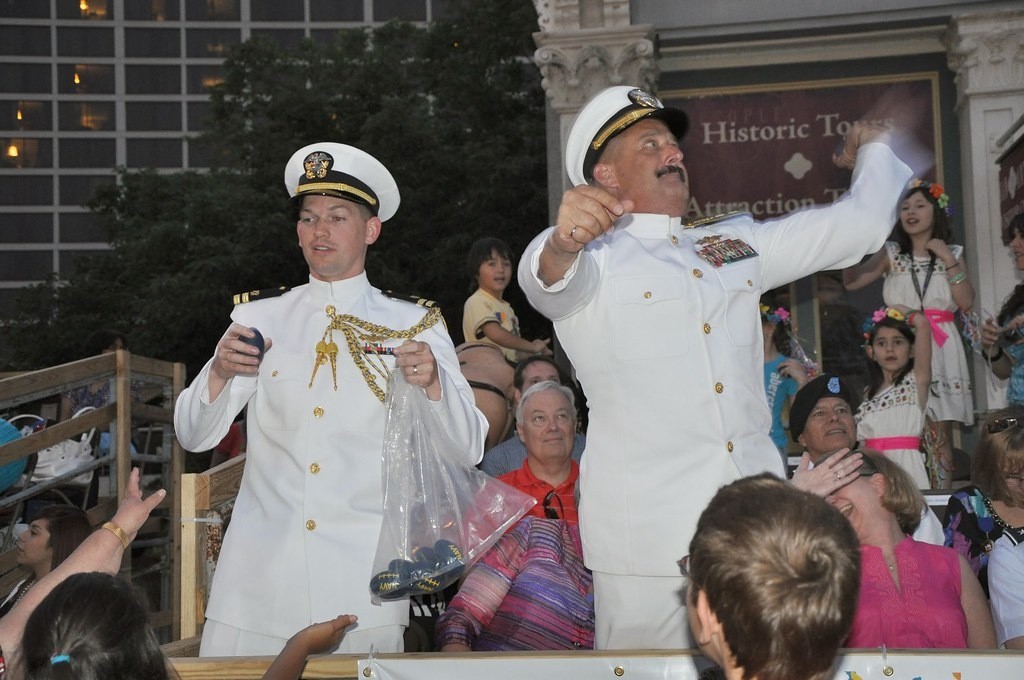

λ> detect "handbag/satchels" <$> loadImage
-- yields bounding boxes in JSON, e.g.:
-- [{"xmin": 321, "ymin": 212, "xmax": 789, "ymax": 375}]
[
  {"xmin": 371, "ymin": 365, "xmax": 539, "ymax": 608},
  {"xmin": 6, "ymin": 406, "xmax": 95, "ymax": 486}
]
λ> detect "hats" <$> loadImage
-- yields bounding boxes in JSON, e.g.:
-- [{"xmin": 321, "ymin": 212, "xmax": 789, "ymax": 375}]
[
  {"xmin": 565, "ymin": 86, "xmax": 690, "ymax": 187},
  {"xmin": 284, "ymin": 142, "xmax": 400, "ymax": 224},
  {"xmin": 790, "ymin": 374, "xmax": 855, "ymax": 442}
]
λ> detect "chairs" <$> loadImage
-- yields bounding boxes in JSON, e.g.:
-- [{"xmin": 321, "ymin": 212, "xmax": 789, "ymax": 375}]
[{"xmin": 11, "ymin": 404, "xmax": 95, "ymax": 515}]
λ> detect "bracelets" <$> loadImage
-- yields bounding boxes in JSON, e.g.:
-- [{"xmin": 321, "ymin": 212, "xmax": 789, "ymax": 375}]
[
  {"xmin": 949, "ymin": 272, "xmax": 967, "ymax": 286},
  {"xmin": 945, "ymin": 262, "xmax": 960, "ymax": 271},
  {"xmin": 102, "ymin": 522, "xmax": 128, "ymax": 548},
  {"xmin": 905, "ymin": 309, "xmax": 923, "ymax": 327},
  {"xmin": 986, "ymin": 348, "xmax": 1003, "ymax": 362}
]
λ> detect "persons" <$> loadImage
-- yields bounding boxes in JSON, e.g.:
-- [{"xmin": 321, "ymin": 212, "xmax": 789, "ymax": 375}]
[
  {"xmin": 456, "ymin": 381, "xmax": 580, "ymax": 591},
  {"xmin": 462, "ymin": 238, "xmax": 553, "ymax": 370},
  {"xmin": 788, "ymin": 179, "xmax": 1024, "ymax": 648},
  {"xmin": 59, "ymin": 330, "xmax": 126, "ymax": 423},
  {"xmin": 677, "ymin": 473, "xmax": 861, "ymax": 680},
  {"xmin": 759, "ymin": 296, "xmax": 821, "ymax": 478},
  {"xmin": 173, "ymin": 142, "xmax": 490, "ymax": 658},
  {"xmin": 517, "ymin": 86, "xmax": 914, "ymax": 651},
  {"xmin": 435, "ymin": 446, "xmax": 864, "ymax": 654},
  {"xmin": 480, "ymin": 357, "xmax": 587, "ymax": 478},
  {"xmin": 0, "ymin": 467, "xmax": 358, "ymax": 680}
]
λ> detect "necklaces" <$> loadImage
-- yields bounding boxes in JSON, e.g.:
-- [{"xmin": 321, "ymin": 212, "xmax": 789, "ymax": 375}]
[
  {"xmin": 887, "ymin": 561, "xmax": 897, "ymax": 572},
  {"xmin": 15, "ymin": 581, "xmax": 34, "ymax": 606}
]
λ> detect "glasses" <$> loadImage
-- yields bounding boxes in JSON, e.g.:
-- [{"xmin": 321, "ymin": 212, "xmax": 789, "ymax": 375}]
[
  {"xmin": 676, "ymin": 554, "xmax": 721, "ymax": 624},
  {"xmin": 983, "ymin": 418, "xmax": 1018, "ymax": 435}
]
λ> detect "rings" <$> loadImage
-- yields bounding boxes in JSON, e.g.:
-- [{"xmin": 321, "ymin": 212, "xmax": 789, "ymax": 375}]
[
  {"xmin": 571, "ymin": 227, "xmax": 576, "ymax": 236},
  {"xmin": 835, "ymin": 473, "xmax": 841, "ymax": 480},
  {"xmin": 413, "ymin": 365, "xmax": 417, "ymax": 375}
]
